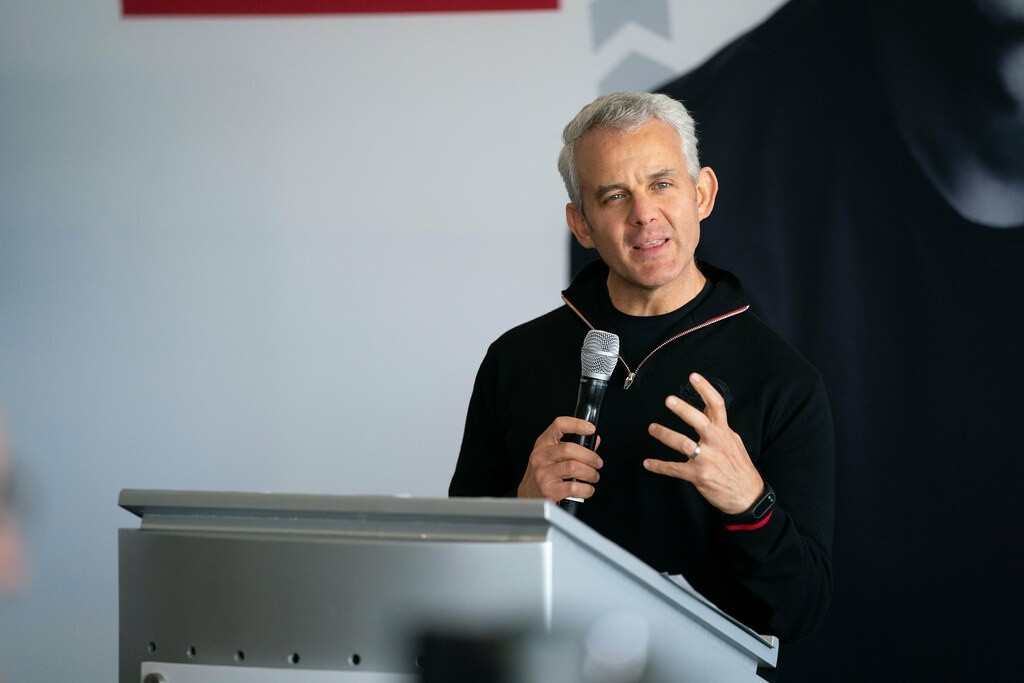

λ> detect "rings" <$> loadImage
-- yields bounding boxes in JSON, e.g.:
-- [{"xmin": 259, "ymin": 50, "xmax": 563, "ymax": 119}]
[{"xmin": 689, "ymin": 442, "xmax": 702, "ymax": 460}]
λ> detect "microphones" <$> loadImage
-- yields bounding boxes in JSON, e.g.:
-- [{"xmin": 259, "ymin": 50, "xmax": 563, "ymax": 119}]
[{"xmin": 560, "ymin": 330, "xmax": 620, "ymax": 522}]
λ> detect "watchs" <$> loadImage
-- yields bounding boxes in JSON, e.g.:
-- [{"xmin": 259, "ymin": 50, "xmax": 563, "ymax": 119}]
[{"xmin": 722, "ymin": 479, "xmax": 776, "ymax": 527}]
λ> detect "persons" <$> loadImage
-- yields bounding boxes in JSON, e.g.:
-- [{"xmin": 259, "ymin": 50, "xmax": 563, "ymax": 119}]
[{"xmin": 447, "ymin": 91, "xmax": 840, "ymax": 636}]
